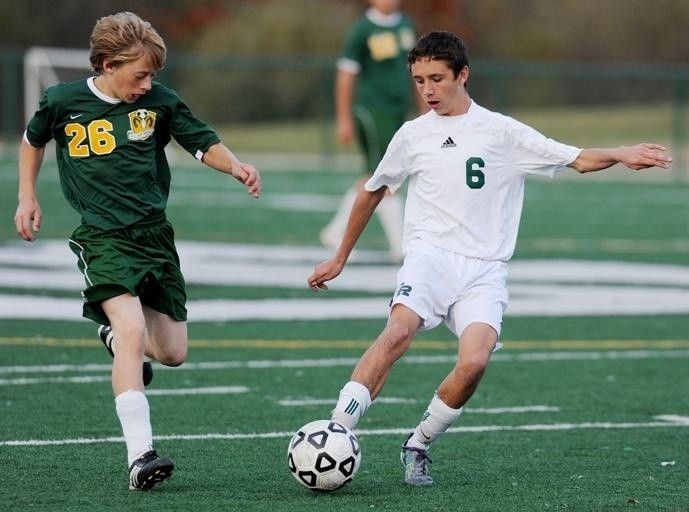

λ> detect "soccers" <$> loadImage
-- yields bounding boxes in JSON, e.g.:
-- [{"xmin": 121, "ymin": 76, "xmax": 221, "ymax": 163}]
[{"xmin": 288, "ymin": 420, "xmax": 362, "ymax": 491}]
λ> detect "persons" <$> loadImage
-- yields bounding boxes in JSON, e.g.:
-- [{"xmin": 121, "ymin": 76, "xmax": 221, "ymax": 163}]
[
  {"xmin": 306, "ymin": 29, "xmax": 674, "ymax": 489},
  {"xmin": 11, "ymin": 11, "xmax": 262, "ymax": 493},
  {"xmin": 322, "ymin": 0, "xmax": 420, "ymax": 265}
]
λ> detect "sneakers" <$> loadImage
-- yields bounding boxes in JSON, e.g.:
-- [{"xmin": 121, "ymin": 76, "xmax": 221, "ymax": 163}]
[
  {"xmin": 128, "ymin": 449, "xmax": 175, "ymax": 491},
  {"xmin": 400, "ymin": 432, "xmax": 434, "ymax": 487},
  {"xmin": 96, "ymin": 322, "xmax": 153, "ymax": 387}
]
